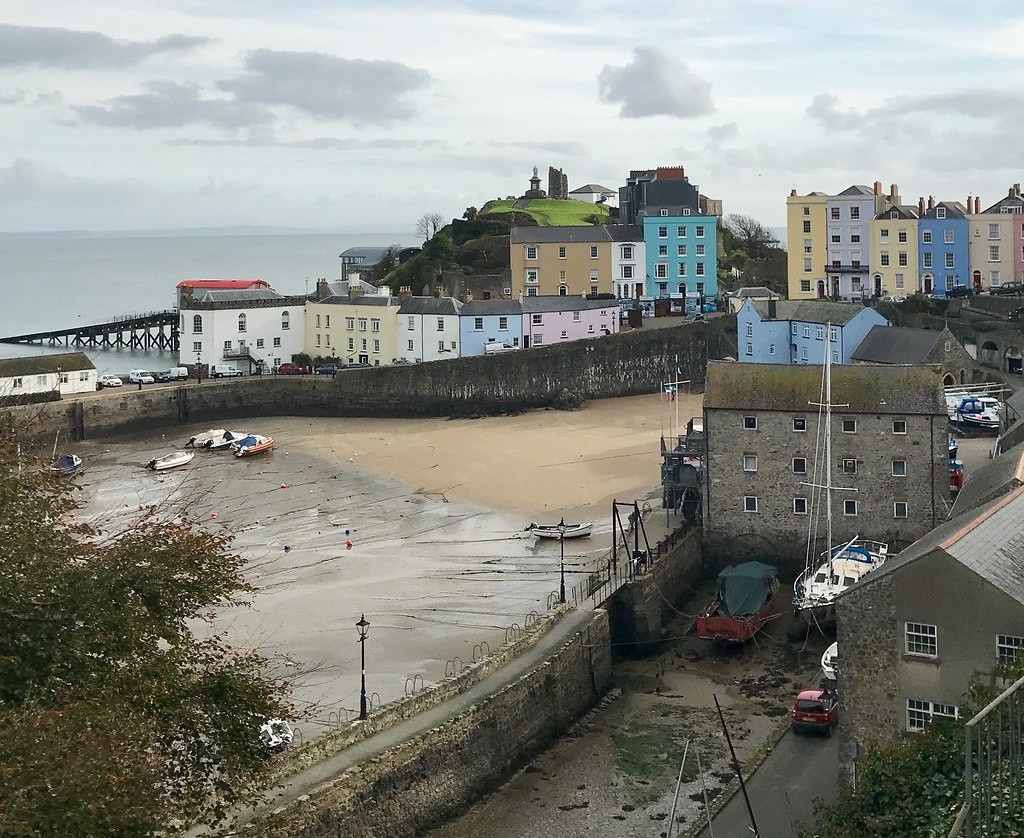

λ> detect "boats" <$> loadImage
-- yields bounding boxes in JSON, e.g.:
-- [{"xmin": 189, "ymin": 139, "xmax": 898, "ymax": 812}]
[
  {"xmin": 949, "ymin": 434, "xmax": 959, "ymax": 459},
  {"xmin": 695, "ymin": 563, "xmax": 782, "ymax": 643},
  {"xmin": 525, "ymin": 522, "xmax": 595, "ymax": 539},
  {"xmin": 145, "ymin": 448, "xmax": 197, "ymax": 469},
  {"xmin": 944, "ymin": 381, "xmax": 1004, "ymax": 431},
  {"xmin": 232, "ymin": 436, "xmax": 275, "ymax": 458},
  {"xmin": 203, "ymin": 428, "xmax": 248, "ymax": 451},
  {"xmin": 187, "ymin": 428, "xmax": 227, "ymax": 447},
  {"xmin": 949, "ymin": 455, "xmax": 966, "ymax": 495},
  {"xmin": 819, "ymin": 641, "xmax": 838, "ymax": 683},
  {"xmin": 50, "ymin": 454, "xmax": 83, "ymax": 476}
]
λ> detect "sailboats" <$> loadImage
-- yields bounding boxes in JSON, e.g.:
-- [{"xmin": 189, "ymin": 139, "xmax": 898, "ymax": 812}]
[{"xmin": 789, "ymin": 317, "xmax": 895, "ymax": 625}]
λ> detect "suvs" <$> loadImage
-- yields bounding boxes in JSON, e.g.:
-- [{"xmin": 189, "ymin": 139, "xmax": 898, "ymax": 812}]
[{"xmin": 945, "ymin": 284, "xmax": 975, "ymax": 299}]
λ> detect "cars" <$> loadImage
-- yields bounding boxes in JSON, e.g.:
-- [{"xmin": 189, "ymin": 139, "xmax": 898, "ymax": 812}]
[
  {"xmin": 278, "ymin": 363, "xmax": 304, "ymax": 375},
  {"xmin": 347, "ymin": 362, "xmax": 363, "ymax": 369},
  {"xmin": 791, "ymin": 689, "xmax": 837, "ymax": 738},
  {"xmin": 98, "ymin": 374, "xmax": 123, "ymax": 386},
  {"xmin": 150, "ymin": 371, "xmax": 171, "ymax": 382},
  {"xmin": 314, "ymin": 363, "xmax": 336, "ymax": 376},
  {"xmin": 991, "ymin": 281, "xmax": 1024, "ymax": 298},
  {"xmin": 681, "ymin": 312, "xmax": 704, "ymax": 324}
]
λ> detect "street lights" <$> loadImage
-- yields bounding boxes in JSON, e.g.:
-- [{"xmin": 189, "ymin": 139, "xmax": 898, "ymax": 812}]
[
  {"xmin": 56, "ymin": 364, "xmax": 62, "ymax": 399},
  {"xmin": 332, "ymin": 347, "xmax": 336, "ymax": 379},
  {"xmin": 196, "ymin": 351, "xmax": 202, "ymax": 385},
  {"xmin": 356, "ymin": 613, "xmax": 369, "ymax": 720},
  {"xmin": 557, "ymin": 516, "xmax": 569, "ymax": 605}
]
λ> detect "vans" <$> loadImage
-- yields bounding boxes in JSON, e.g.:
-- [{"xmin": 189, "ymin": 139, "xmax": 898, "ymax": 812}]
[
  {"xmin": 130, "ymin": 369, "xmax": 154, "ymax": 384},
  {"xmin": 164, "ymin": 367, "xmax": 188, "ymax": 382},
  {"xmin": 484, "ymin": 343, "xmax": 520, "ymax": 356},
  {"xmin": 210, "ymin": 365, "xmax": 244, "ymax": 378}
]
